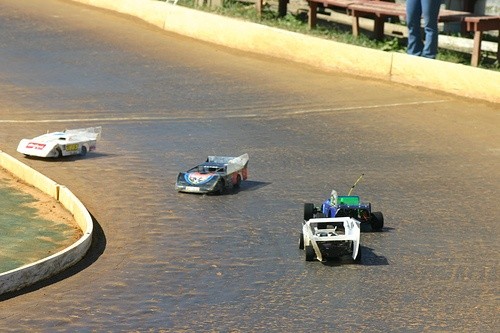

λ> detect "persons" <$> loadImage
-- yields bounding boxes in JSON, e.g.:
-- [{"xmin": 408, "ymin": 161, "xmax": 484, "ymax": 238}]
[{"xmin": 406, "ymin": 0, "xmax": 442, "ymax": 59}]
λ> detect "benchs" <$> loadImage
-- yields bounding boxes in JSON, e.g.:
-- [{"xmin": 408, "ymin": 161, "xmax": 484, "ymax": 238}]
[
  {"xmin": 459, "ymin": 14, "xmax": 500, "ymax": 69},
  {"xmin": 251, "ymin": 0, "xmax": 471, "ymax": 52}
]
